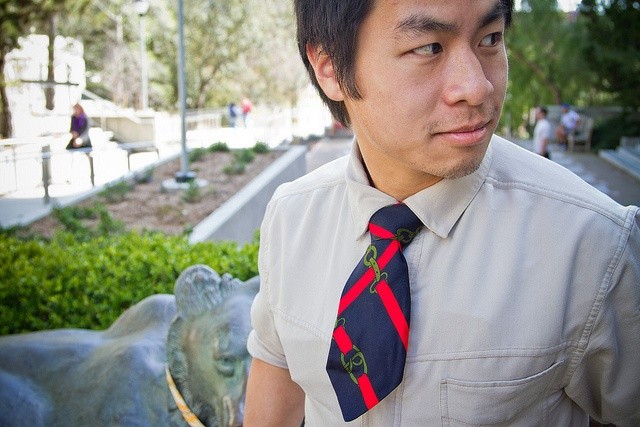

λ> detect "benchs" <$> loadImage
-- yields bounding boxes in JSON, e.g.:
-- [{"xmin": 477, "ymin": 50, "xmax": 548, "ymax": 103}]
[{"xmin": 566, "ymin": 113, "xmax": 594, "ymax": 153}]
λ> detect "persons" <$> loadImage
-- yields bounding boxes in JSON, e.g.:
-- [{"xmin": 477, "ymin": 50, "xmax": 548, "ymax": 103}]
[
  {"xmin": 559, "ymin": 106, "xmax": 581, "ymax": 149},
  {"xmin": 228, "ymin": 103, "xmax": 237, "ymax": 123},
  {"xmin": 534, "ymin": 105, "xmax": 551, "ymax": 157},
  {"xmin": 66, "ymin": 103, "xmax": 92, "ymax": 148},
  {"xmin": 241, "ymin": 0, "xmax": 639, "ymax": 427},
  {"xmin": 241, "ymin": 104, "xmax": 250, "ymax": 127}
]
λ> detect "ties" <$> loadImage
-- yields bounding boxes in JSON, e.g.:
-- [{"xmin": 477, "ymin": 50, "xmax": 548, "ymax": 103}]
[{"xmin": 326, "ymin": 204, "xmax": 423, "ymax": 421}]
